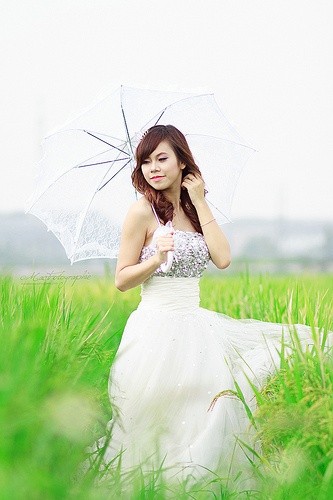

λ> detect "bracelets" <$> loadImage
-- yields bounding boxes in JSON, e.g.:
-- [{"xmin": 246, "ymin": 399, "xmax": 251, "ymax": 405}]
[{"xmin": 200, "ymin": 218, "xmax": 217, "ymax": 226}]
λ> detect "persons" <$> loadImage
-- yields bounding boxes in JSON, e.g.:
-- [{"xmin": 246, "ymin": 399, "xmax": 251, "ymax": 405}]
[{"xmin": 79, "ymin": 124, "xmax": 332, "ymax": 499}]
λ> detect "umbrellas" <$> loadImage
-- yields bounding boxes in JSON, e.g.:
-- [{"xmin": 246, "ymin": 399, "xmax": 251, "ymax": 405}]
[{"xmin": 23, "ymin": 83, "xmax": 258, "ymax": 273}]
